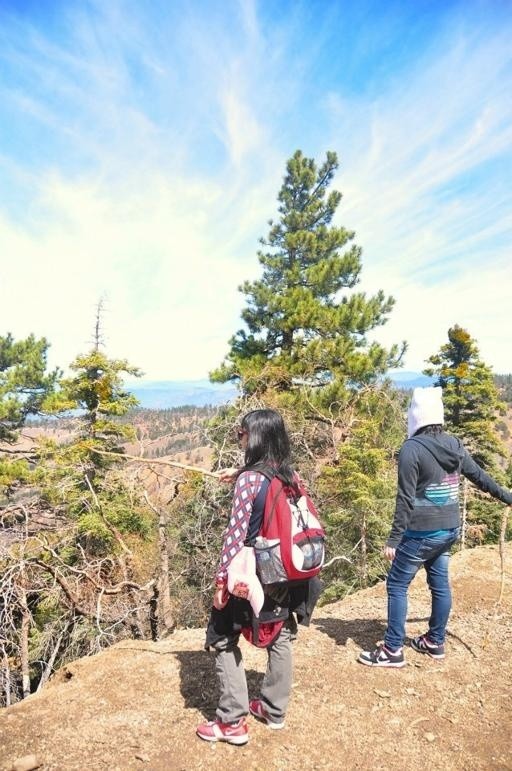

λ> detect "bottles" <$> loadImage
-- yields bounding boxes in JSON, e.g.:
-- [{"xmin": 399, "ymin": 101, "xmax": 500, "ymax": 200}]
[{"xmin": 253, "ymin": 535, "xmax": 279, "ymax": 585}]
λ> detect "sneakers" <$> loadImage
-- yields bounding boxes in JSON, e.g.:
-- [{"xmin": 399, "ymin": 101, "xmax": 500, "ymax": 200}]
[
  {"xmin": 249, "ymin": 696, "xmax": 285, "ymax": 731},
  {"xmin": 195, "ymin": 716, "xmax": 249, "ymax": 746},
  {"xmin": 410, "ymin": 633, "xmax": 445, "ymax": 659},
  {"xmin": 358, "ymin": 644, "xmax": 406, "ymax": 668}
]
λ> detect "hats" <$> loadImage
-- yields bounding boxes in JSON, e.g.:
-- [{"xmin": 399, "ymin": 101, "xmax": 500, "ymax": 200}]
[
  {"xmin": 226, "ymin": 547, "xmax": 265, "ymax": 618},
  {"xmin": 406, "ymin": 386, "xmax": 444, "ymax": 439}
]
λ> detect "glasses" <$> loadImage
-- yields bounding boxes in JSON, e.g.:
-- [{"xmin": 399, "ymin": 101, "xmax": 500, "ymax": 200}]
[{"xmin": 237, "ymin": 430, "xmax": 249, "ymax": 440}]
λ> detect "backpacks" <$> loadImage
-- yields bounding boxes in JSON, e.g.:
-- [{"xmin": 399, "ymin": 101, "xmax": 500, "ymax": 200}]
[{"xmin": 246, "ymin": 463, "xmax": 328, "ymax": 588}]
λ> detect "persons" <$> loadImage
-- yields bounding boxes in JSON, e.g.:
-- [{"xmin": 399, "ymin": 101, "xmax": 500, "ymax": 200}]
[
  {"xmin": 357, "ymin": 382, "xmax": 512, "ymax": 668},
  {"xmin": 197, "ymin": 409, "xmax": 307, "ymax": 746}
]
